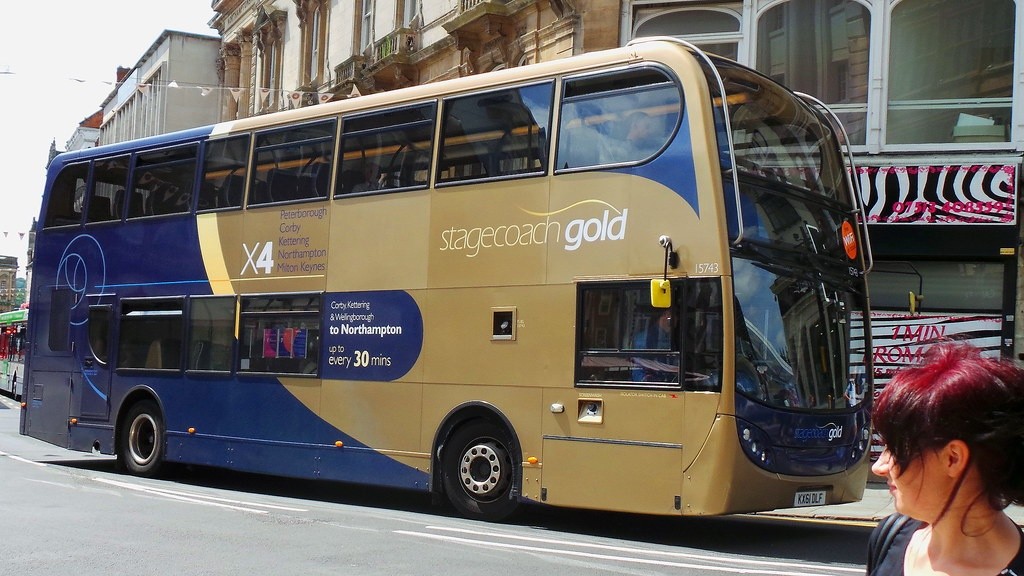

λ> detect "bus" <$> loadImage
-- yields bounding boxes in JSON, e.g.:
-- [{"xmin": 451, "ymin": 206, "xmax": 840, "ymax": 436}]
[
  {"xmin": 0, "ymin": 309, "xmax": 29, "ymax": 396},
  {"xmin": 20, "ymin": 35, "xmax": 924, "ymax": 525}
]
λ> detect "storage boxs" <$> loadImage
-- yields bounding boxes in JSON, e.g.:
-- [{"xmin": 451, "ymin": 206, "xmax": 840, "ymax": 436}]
[{"xmin": 947, "ymin": 124, "xmax": 1012, "ymax": 143}]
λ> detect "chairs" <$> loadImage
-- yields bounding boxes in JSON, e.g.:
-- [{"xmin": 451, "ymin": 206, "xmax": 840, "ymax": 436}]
[
  {"xmin": 94, "ymin": 326, "xmax": 317, "ymax": 376},
  {"xmin": 84, "ymin": 141, "xmax": 407, "ymax": 226}
]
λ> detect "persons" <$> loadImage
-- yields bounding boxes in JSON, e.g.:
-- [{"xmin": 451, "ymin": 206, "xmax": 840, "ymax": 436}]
[
  {"xmin": 633, "ymin": 311, "xmax": 678, "ymax": 382},
  {"xmin": 845, "ymin": 372, "xmax": 856, "ymax": 406},
  {"xmin": 616, "ymin": 112, "xmax": 649, "ymax": 160},
  {"xmin": 351, "ymin": 163, "xmax": 383, "ymax": 193},
  {"xmin": 866, "ymin": 343, "xmax": 1024, "ymax": 576}
]
